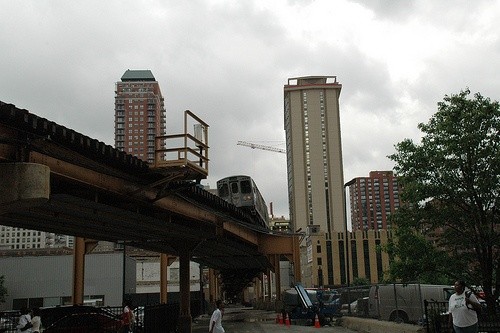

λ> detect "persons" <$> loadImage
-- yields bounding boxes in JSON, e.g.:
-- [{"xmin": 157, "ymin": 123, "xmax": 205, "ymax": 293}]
[
  {"xmin": 121, "ymin": 302, "xmax": 133, "ymax": 322},
  {"xmin": 447, "ymin": 279, "xmax": 482, "ymax": 333},
  {"xmin": 17, "ymin": 307, "xmax": 32, "ymax": 333},
  {"xmin": 20, "ymin": 307, "xmax": 43, "ymax": 333},
  {"xmin": 208, "ymin": 298, "xmax": 226, "ymax": 333}
]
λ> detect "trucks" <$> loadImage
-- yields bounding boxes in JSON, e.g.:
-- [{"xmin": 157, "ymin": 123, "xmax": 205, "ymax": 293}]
[{"xmin": 266, "ymin": 280, "xmax": 458, "ymax": 323}]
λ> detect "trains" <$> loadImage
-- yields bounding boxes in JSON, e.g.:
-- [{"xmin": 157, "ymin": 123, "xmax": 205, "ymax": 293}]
[{"xmin": 216, "ymin": 175, "xmax": 268, "ymax": 227}]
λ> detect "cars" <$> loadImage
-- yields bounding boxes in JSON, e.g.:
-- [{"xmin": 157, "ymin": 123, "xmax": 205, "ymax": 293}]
[{"xmin": 0, "ymin": 304, "xmax": 146, "ymax": 333}]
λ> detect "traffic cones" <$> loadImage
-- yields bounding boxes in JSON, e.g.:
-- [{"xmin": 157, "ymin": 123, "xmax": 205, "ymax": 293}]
[
  {"xmin": 277, "ymin": 312, "xmax": 291, "ymax": 326},
  {"xmin": 313, "ymin": 314, "xmax": 321, "ymax": 328}
]
